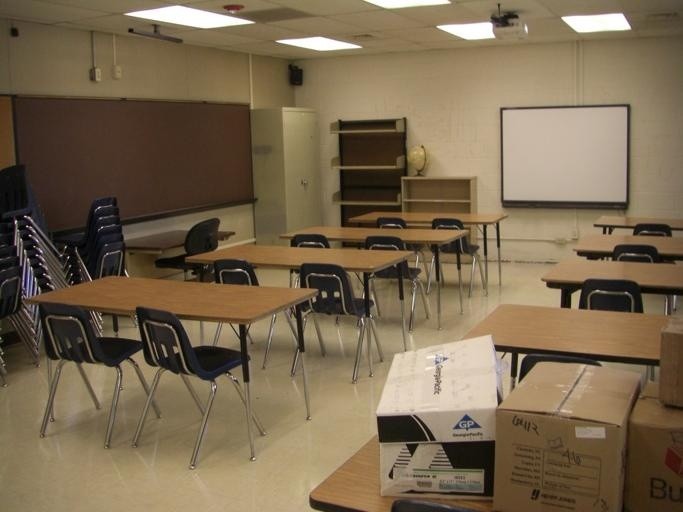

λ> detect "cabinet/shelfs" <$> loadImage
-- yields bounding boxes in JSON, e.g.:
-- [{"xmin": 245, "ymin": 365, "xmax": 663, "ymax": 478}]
[
  {"xmin": 243, "ymin": 105, "xmax": 323, "ymax": 256},
  {"xmin": 398, "ymin": 171, "xmax": 478, "ymax": 251},
  {"xmin": 325, "ymin": 115, "xmax": 408, "ymax": 248}
]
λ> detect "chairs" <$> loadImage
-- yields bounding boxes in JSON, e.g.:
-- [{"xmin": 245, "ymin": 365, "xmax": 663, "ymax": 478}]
[
  {"xmin": 126, "ymin": 305, "xmax": 269, "ymax": 471},
  {"xmin": 577, "ymin": 278, "xmax": 644, "ymax": 315},
  {"xmin": 610, "ymin": 244, "xmax": 660, "ymax": 265},
  {"xmin": 517, "ymin": 350, "xmax": 602, "ymax": 386},
  {"xmin": 0, "ymin": 158, "xmax": 131, "ymax": 386},
  {"xmin": 433, "ymin": 217, "xmax": 488, "ymax": 288},
  {"xmin": 632, "ymin": 222, "xmax": 672, "ymax": 238},
  {"xmin": 286, "ymin": 261, "xmax": 385, "ymax": 385},
  {"xmin": 205, "ymin": 258, "xmax": 280, "ymax": 373},
  {"xmin": 360, "ymin": 236, "xmax": 431, "ymax": 333},
  {"xmin": 31, "ymin": 300, "xmax": 161, "ymax": 450},
  {"xmin": 388, "ymin": 496, "xmax": 488, "ymax": 512},
  {"xmin": 287, "ymin": 232, "xmax": 332, "ymax": 318},
  {"xmin": 369, "ymin": 216, "xmax": 429, "ymax": 291}
]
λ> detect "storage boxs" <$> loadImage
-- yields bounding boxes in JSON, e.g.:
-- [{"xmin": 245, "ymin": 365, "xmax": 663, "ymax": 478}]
[
  {"xmin": 653, "ymin": 312, "xmax": 683, "ymax": 412},
  {"xmin": 371, "ymin": 333, "xmax": 507, "ymax": 502},
  {"xmin": 622, "ymin": 377, "xmax": 681, "ymax": 512},
  {"xmin": 488, "ymin": 359, "xmax": 643, "ymax": 512}
]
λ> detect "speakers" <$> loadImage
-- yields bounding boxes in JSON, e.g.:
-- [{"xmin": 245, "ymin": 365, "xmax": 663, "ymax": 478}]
[{"xmin": 291, "ymin": 69, "xmax": 303, "ymax": 85}]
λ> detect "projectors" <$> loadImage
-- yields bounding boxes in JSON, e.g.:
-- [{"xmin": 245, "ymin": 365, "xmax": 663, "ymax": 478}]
[{"xmin": 493, "ymin": 22, "xmax": 528, "ymax": 40}]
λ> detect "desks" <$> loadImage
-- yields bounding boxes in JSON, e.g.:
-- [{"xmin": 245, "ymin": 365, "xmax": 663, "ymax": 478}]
[
  {"xmin": 459, "ymin": 300, "xmax": 681, "ymax": 381},
  {"xmin": 459, "ymin": 302, "xmax": 683, "ymax": 366},
  {"xmin": 572, "ymin": 230, "xmax": 683, "ymax": 264},
  {"xmin": 306, "ymin": 425, "xmax": 493, "ymax": 512},
  {"xmin": 16, "ymin": 272, "xmax": 323, "ymax": 466},
  {"xmin": 280, "ymin": 225, "xmax": 471, "ymax": 334},
  {"xmin": 185, "ymin": 243, "xmax": 416, "ymax": 378},
  {"xmin": 592, "ymin": 213, "xmax": 683, "ymax": 230},
  {"xmin": 540, "ymin": 255, "xmax": 683, "ymax": 313},
  {"xmin": 346, "ymin": 210, "xmax": 510, "ymax": 297}
]
